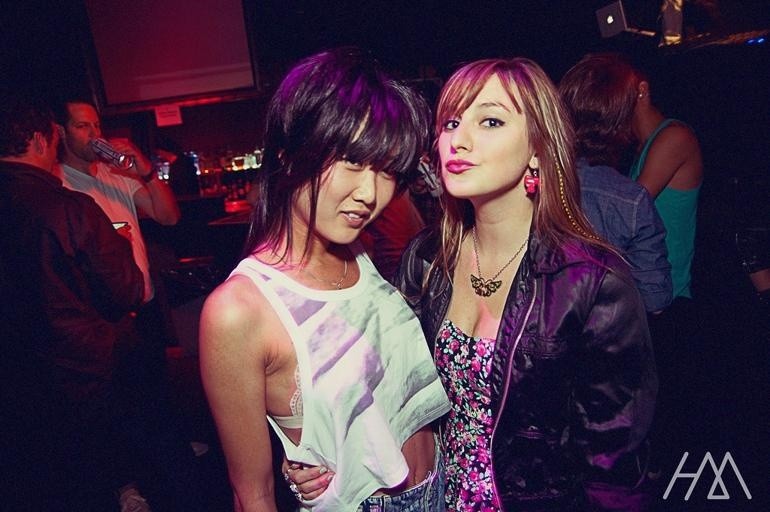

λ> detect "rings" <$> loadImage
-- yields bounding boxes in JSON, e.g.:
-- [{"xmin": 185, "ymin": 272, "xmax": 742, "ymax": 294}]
[
  {"xmin": 282, "ymin": 468, "xmax": 295, "ymax": 484},
  {"xmin": 295, "ymin": 491, "xmax": 304, "ymax": 503},
  {"xmin": 290, "ymin": 485, "xmax": 298, "ymax": 494}
]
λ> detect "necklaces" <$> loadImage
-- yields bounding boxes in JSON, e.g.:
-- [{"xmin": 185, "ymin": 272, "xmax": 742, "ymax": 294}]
[
  {"xmin": 274, "ymin": 241, "xmax": 355, "ymax": 292},
  {"xmin": 468, "ymin": 222, "xmax": 532, "ymax": 298}
]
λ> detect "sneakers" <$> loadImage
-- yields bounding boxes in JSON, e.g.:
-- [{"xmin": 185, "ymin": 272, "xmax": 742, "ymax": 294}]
[
  {"xmin": 120, "ymin": 488, "xmax": 150, "ymax": 512},
  {"xmin": 177, "ymin": 439, "xmax": 208, "ymax": 461}
]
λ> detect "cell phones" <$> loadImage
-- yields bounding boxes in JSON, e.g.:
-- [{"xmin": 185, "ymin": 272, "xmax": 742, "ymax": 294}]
[{"xmin": 111, "ymin": 221, "xmax": 128, "ymax": 230}]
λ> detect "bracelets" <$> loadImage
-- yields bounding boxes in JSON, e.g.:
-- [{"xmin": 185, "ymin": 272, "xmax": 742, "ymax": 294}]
[{"xmin": 140, "ymin": 164, "xmax": 157, "ymax": 185}]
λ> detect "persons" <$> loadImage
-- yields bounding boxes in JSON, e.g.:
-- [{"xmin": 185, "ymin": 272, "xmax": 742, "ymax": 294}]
[
  {"xmin": 50, "ymin": 94, "xmax": 185, "ymax": 325},
  {"xmin": 616, "ymin": 54, "xmax": 714, "ymax": 511},
  {"xmin": 192, "ymin": 45, "xmax": 454, "ymax": 512},
  {"xmin": 152, "ymin": 131, "xmax": 200, "ymax": 198},
  {"xmin": 0, "ymin": 83, "xmax": 153, "ymax": 510},
  {"xmin": 547, "ymin": 54, "xmax": 676, "ymax": 322},
  {"xmin": 727, "ymin": 200, "xmax": 770, "ymax": 313},
  {"xmin": 279, "ymin": 53, "xmax": 660, "ymax": 512}
]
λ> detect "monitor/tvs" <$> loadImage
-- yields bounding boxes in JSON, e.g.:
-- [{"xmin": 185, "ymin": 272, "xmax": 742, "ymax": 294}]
[{"xmin": 72, "ymin": 0, "xmax": 261, "ymax": 130}]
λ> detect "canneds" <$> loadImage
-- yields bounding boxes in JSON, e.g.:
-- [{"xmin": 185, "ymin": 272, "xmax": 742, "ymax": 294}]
[
  {"xmin": 415, "ymin": 153, "xmax": 443, "ymax": 198},
  {"xmin": 90, "ymin": 137, "xmax": 134, "ymax": 170}
]
